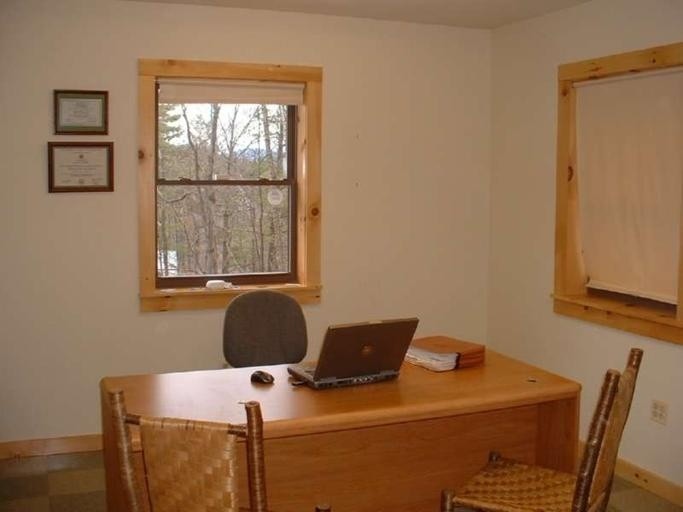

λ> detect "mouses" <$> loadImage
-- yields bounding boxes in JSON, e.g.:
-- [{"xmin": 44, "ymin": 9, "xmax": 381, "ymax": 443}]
[{"xmin": 250, "ymin": 369, "xmax": 276, "ymax": 385}]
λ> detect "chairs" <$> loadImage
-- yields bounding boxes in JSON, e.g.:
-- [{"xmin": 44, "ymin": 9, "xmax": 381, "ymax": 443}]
[
  {"xmin": 98, "ymin": 336, "xmax": 583, "ymax": 512},
  {"xmin": 441, "ymin": 347, "xmax": 644, "ymax": 512},
  {"xmin": 223, "ymin": 291, "xmax": 308, "ymax": 368},
  {"xmin": 108, "ymin": 388, "xmax": 266, "ymax": 511}
]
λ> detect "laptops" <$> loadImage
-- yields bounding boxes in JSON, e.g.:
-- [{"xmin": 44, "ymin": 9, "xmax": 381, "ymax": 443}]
[{"xmin": 287, "ymin": 316, "xmax": 420, "ymax": 390}]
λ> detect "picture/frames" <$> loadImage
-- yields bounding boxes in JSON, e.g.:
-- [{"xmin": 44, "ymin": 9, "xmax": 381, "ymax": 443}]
[
  {"xmin": 47, "ymin": 141, "xmax": 115, "ymax": 193},
  {"xmin": 53, "ymin": 88, "xmax": 109, "ymax": 134}
]
